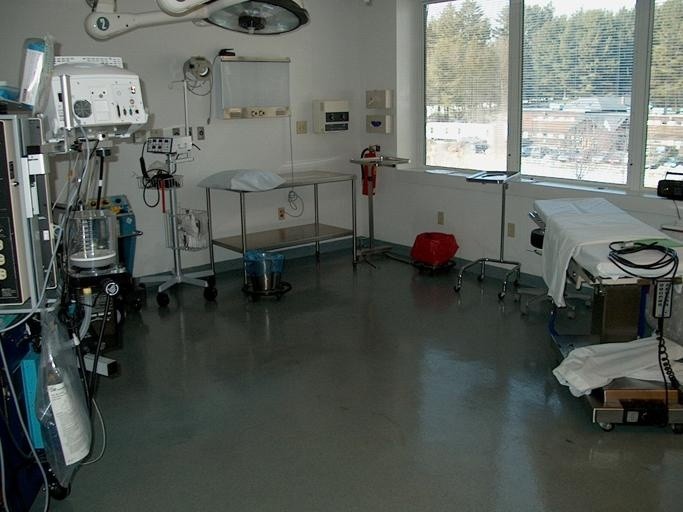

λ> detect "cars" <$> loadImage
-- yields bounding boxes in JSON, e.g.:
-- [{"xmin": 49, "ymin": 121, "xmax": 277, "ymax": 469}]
[
  {"xmin": 429, "ymin": 137, "xmax": 458, "ymax": 152},
  {"xmin": 647, "ymin": 106, "xmax": 682, "ymax": 126},
  {"xmin": 521, "ymin": 137, "xmax": 682, "ymax": 171},
  {"xmin": 456, "ymin": 136, "xmax": 490, "ymax": 155}
]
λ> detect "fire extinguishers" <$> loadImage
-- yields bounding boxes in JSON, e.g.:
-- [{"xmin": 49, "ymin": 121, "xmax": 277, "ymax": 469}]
[{"xmin": 361, "ymin": 144, "xmax": 380, "ymax": 196}]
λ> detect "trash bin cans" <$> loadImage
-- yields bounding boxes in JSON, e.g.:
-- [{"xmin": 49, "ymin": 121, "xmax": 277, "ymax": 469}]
[
  {"xmin": 410, "ymin": 232, "xmax": 456, "ymax": 277},
  {"xmin": 241, "ymin": 251, "xmax": 292, "ymax": 302}
]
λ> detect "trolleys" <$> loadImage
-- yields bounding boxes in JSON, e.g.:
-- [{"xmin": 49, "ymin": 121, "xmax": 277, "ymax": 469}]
[{"xmin": 450, "ymin": 167, "xmax": 523, "ymax": 301}]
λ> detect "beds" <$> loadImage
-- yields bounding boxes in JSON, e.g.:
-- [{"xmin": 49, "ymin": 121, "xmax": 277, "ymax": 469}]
[{"xmin": 527, "ymin": 196, "xmax": 683, "ymax": 433}]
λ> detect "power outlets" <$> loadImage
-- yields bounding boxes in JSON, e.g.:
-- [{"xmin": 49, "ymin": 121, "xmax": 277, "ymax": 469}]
[{"xmin": 277, "ymin": 207, "xmax": 286, "ymax": 220}]
